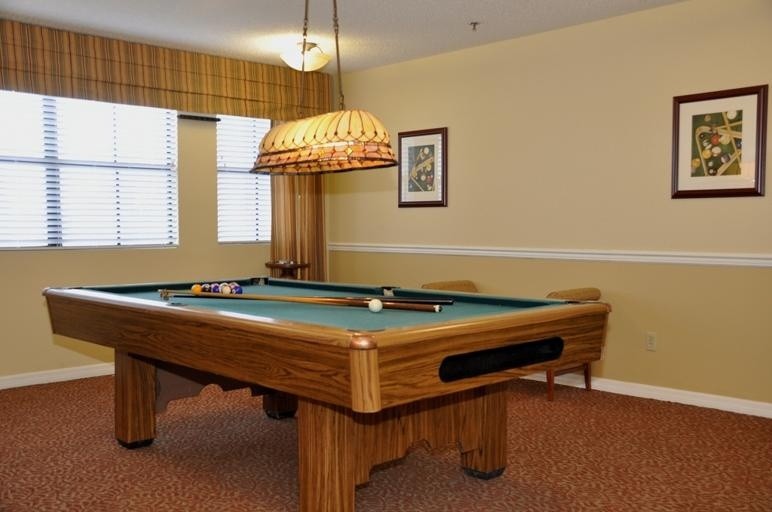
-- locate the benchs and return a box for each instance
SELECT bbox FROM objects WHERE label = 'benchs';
[423,280,600,401]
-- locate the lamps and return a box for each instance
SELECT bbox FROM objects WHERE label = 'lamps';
[249,0,399,174]
[280,43,331,72]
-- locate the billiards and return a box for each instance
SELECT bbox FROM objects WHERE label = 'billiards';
[191,282,244,297]
[691,110,741,175]
[369,299,382,314]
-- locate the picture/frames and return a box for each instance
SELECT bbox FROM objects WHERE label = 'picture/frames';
[671,84,768,198]
[398,127,447,207]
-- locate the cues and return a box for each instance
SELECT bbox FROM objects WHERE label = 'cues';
[157,287,442,313]
[160,291,454,306]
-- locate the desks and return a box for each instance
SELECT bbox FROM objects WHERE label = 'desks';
[43,276,612,512]
[265,259,310,279]
